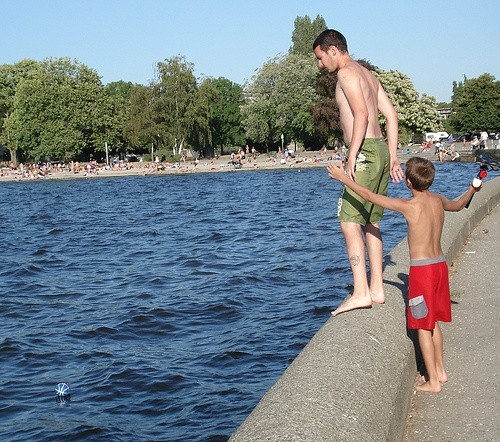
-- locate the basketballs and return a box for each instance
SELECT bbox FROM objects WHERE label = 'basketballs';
[55,383,70,396]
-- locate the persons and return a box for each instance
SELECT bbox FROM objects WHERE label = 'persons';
[0,153,202,181]
[207,143,347,169]
[408,131,500,160]
[313,29,405,316]
[326,157,478,392]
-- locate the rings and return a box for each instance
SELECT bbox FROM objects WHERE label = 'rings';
[396,170,399,172]
